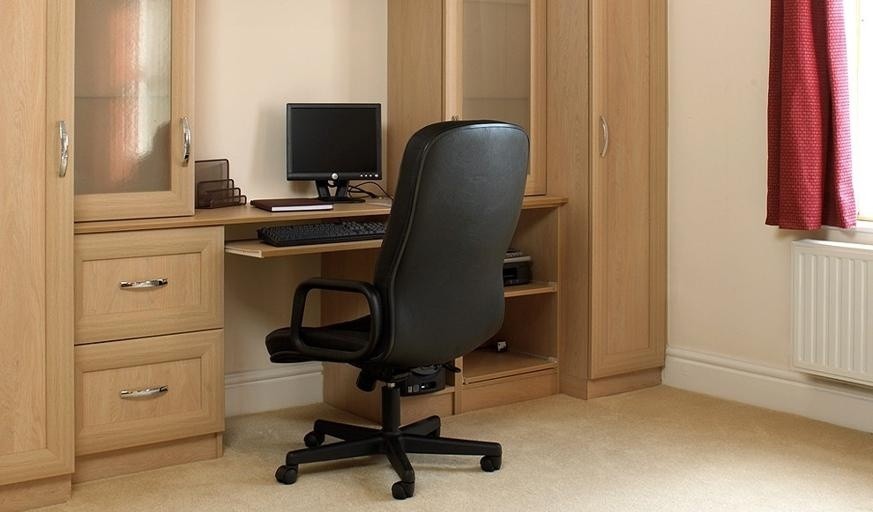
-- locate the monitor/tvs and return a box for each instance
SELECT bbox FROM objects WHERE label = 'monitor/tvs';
[286,102,382,203]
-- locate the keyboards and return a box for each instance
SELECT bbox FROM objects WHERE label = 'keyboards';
[258,222,387,247]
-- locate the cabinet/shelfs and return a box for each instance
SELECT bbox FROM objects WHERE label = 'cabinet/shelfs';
[1,2,73,493]
[547,1,667,400]
[75,0,198,221]
[389,1,550,199]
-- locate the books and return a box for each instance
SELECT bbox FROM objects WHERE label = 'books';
[250,197,333,212]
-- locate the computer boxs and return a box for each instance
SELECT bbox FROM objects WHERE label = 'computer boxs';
[396,362,446,396]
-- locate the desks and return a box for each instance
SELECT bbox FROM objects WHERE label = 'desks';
[69,191,567,489]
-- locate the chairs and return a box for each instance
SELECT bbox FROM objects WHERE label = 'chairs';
[266,122,532,496]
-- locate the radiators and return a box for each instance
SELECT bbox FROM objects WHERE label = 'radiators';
[791,234,872,394]
